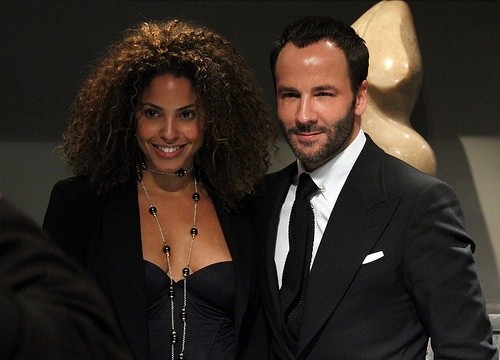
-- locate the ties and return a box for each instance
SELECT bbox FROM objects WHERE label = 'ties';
[280,173,320,340]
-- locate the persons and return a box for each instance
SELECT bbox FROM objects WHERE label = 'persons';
[233,15,497,360]
[41,16,262,360]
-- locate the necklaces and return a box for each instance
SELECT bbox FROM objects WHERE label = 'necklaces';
[131,163,200,360]
